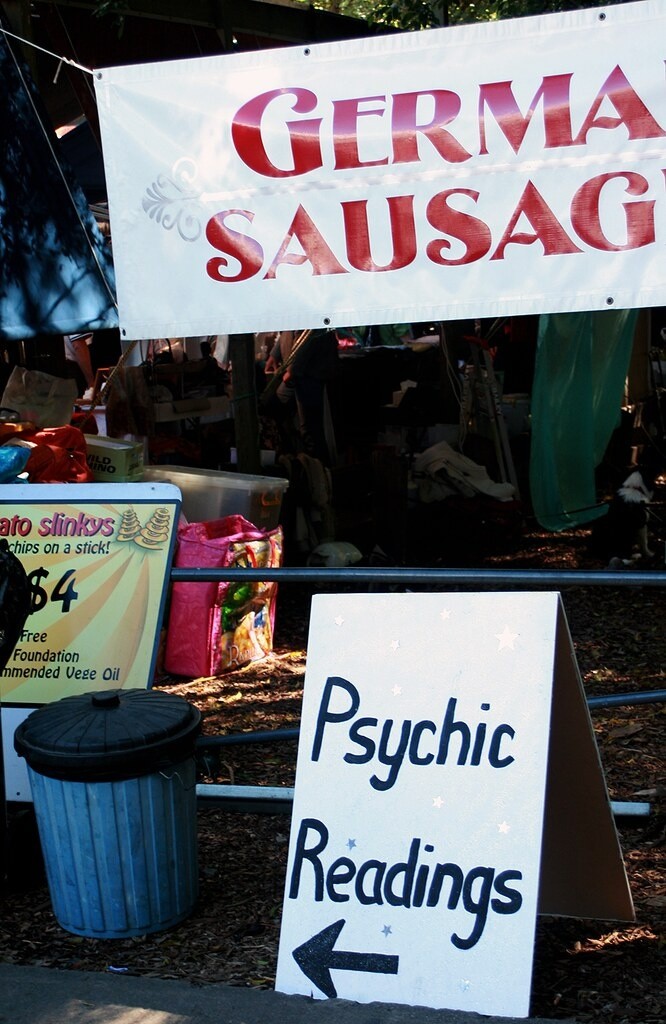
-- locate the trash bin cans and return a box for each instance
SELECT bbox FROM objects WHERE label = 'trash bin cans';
[13,688,209,939]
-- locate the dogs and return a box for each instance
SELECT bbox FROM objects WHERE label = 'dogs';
[581,464,664,569]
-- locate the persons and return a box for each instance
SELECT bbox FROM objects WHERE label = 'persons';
[262,329,333,470]
[441,319,493,406]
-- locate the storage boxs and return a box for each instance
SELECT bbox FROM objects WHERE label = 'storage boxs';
[76,432,145,483]
[141,464,291,531]
[499,399,530,431]
